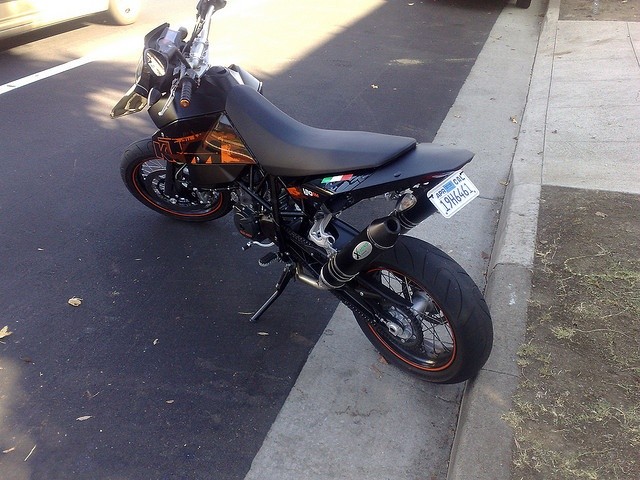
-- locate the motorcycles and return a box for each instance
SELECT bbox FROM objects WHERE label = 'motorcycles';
[109,0,492,384]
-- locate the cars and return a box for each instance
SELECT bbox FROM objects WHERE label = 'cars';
[0,1,143,40]
